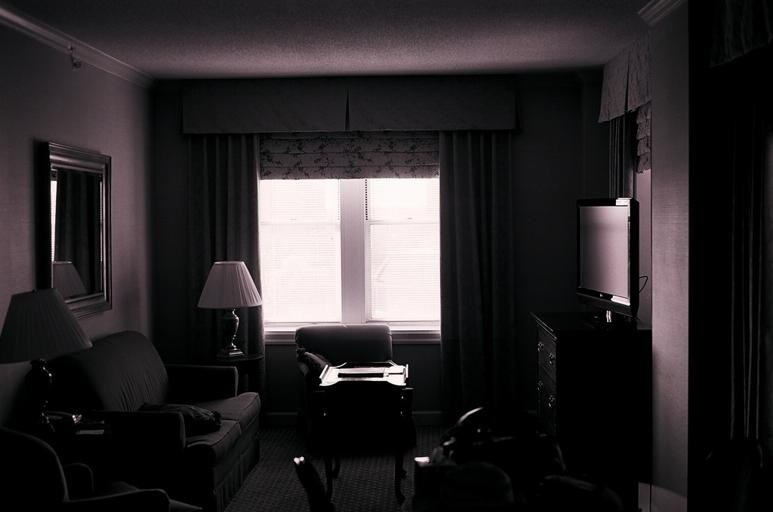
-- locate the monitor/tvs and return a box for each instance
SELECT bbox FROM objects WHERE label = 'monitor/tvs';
[573,197,640,328]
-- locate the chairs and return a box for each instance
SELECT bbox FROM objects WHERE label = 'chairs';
[291,322,419,450]
[291,455,341,511]
[0,427,205,512]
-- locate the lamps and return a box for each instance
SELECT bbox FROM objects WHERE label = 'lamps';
[51,257,89,297]
[191,260,264,357]
[0,283,95,437]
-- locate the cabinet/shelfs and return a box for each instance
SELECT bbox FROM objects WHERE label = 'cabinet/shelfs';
[524,302,652,462]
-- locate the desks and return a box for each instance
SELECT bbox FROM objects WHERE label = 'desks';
[43,423,112,473]
[200,356,268,425]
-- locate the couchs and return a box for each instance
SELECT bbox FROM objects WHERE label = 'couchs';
[71,325,267,512]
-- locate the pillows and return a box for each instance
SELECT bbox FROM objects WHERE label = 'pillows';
[137,398,222,435]
[294,345,328,372]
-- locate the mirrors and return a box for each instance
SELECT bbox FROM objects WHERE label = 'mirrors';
[37,135,115,322]
